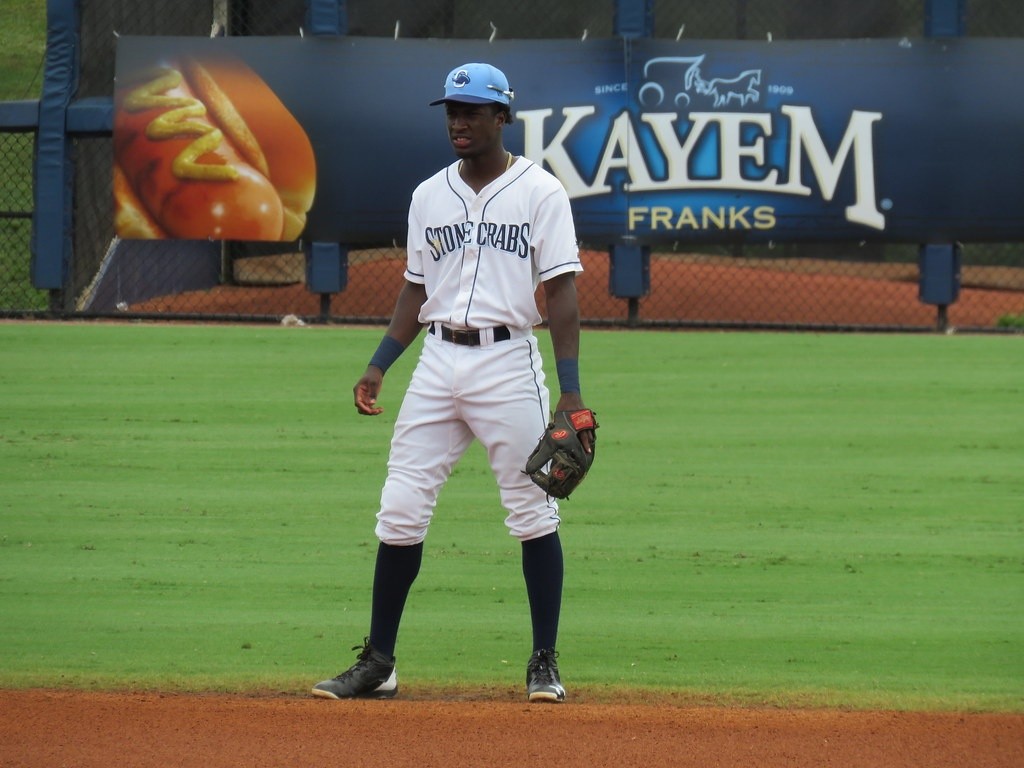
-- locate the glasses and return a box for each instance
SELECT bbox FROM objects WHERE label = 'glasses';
[487,84,514,101]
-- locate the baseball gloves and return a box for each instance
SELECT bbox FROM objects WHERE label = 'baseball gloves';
[519,408,601,505]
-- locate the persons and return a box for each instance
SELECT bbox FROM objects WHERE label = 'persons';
[310,63,600,706]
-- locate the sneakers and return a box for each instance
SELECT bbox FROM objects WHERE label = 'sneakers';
[311,636,398,700]
[526,644,566,703]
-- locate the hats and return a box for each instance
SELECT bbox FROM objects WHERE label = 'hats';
[429,63,509,108]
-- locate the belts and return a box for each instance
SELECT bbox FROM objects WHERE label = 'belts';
[429,321,510,345]
